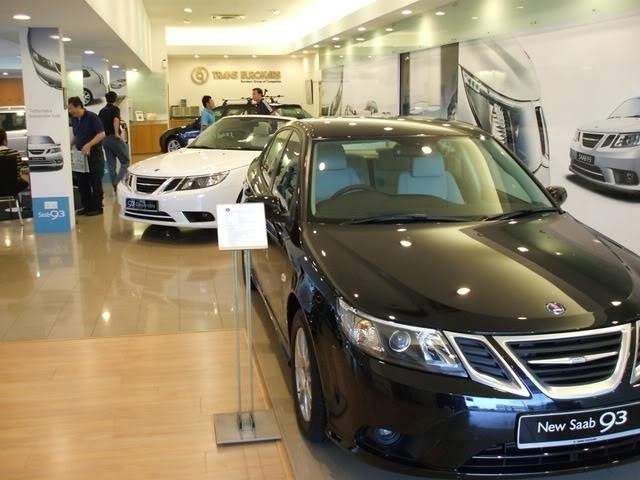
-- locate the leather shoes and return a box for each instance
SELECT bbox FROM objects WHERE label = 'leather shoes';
[77,208,87,215]
[87,208,103,216]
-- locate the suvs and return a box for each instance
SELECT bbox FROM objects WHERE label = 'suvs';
[0,104,29,165]
[158,89,315,156]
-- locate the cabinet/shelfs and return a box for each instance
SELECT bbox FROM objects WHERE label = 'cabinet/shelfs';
[129,117,195,155]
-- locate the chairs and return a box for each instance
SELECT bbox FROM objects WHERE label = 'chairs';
[308,141,469,214]
[246,121,276,149]
[0,153,29,226]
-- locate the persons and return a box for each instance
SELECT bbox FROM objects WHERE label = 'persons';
[252,88,274,116]
[0,129,29,194]
[68,96,105,216]
[98,92,130,192]
[199,96,214,132]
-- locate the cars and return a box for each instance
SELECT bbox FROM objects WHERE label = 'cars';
[320,63,343,117]
[232,112,639,479]
[26,27,62,91]
[110,78,126,89]
[83,62,106,107]
[456,37,551,187]
[565,93,640,193]
[110,113,297,238]
[26,135,66,173]
